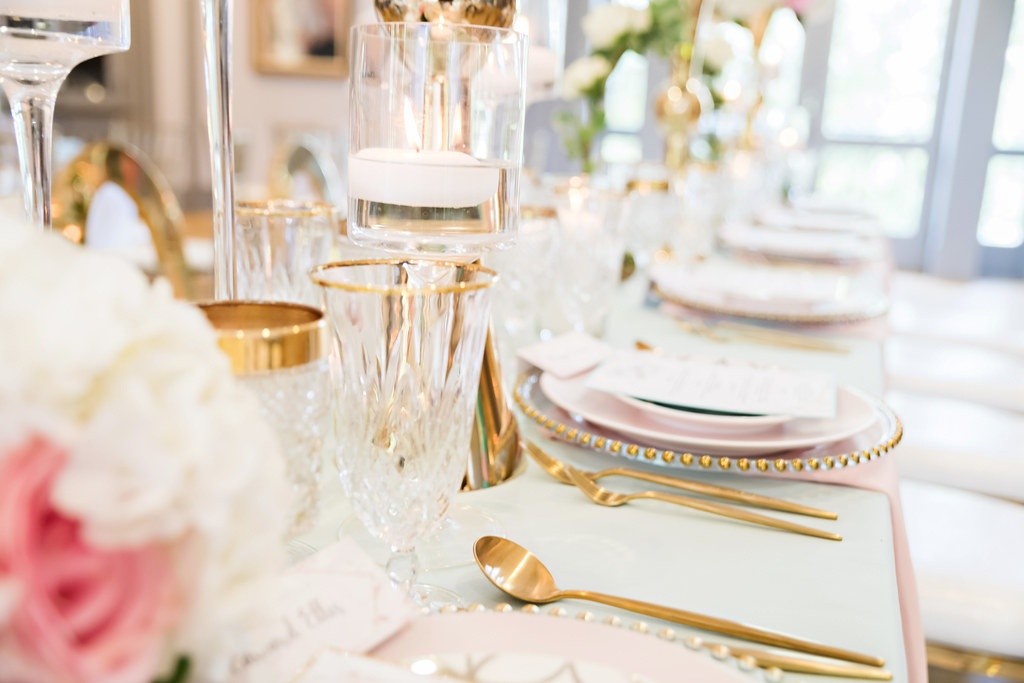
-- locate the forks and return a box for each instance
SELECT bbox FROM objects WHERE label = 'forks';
[562,462,844,542]
[513,437,836,520]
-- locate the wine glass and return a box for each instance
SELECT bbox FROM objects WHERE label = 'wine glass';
[1,0,131,238]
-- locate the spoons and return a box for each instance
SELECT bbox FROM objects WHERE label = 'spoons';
[472,536,885,667]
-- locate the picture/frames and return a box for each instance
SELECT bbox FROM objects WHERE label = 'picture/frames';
[249,0,352,79]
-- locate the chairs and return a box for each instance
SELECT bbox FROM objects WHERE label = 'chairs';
[885,270,1024,683]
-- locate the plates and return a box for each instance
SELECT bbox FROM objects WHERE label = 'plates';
[512,365,904,473]
[617,392,791,436]
[650,204,884,322]
[372,602,784,682]
[536,370,881,457]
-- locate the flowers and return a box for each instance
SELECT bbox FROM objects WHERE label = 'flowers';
[0,212,286,683]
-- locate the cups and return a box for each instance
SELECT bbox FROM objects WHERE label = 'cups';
[346,20,529,254]
[213,195,342,315]
[313,252,498,611]
[195,295,328,542]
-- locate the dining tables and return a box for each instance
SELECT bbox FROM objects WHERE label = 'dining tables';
[387,219,930,683]
